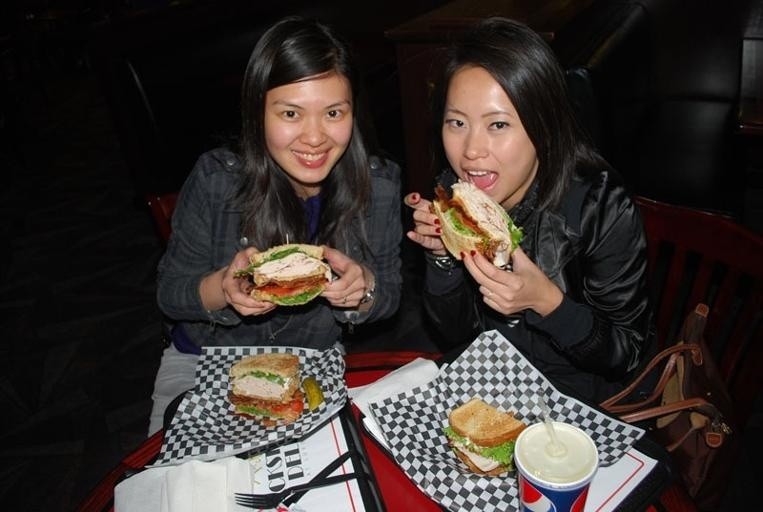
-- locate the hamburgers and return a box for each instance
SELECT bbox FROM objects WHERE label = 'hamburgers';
[427,178,527,271]
[236,242,335,308]
[225,352,305,428]
[445,397,528,478]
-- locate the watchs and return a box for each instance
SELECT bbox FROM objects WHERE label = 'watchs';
[357,261,376,315]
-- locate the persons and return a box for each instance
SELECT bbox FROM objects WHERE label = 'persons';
[403,14,661,448]
[155,18,406,438]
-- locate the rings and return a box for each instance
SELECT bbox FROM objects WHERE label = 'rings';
[342,296,347,305]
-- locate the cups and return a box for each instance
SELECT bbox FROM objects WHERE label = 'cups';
[512,419,600,512]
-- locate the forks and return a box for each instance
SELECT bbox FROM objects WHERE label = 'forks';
[234,469,372,509]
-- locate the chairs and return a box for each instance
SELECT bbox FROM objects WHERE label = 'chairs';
[630,192,763,510]
[145,191,181,245]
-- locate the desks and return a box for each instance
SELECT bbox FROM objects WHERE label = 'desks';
[386,0,594,202]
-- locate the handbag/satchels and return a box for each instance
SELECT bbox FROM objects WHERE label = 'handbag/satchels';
[656,304,733,501]
